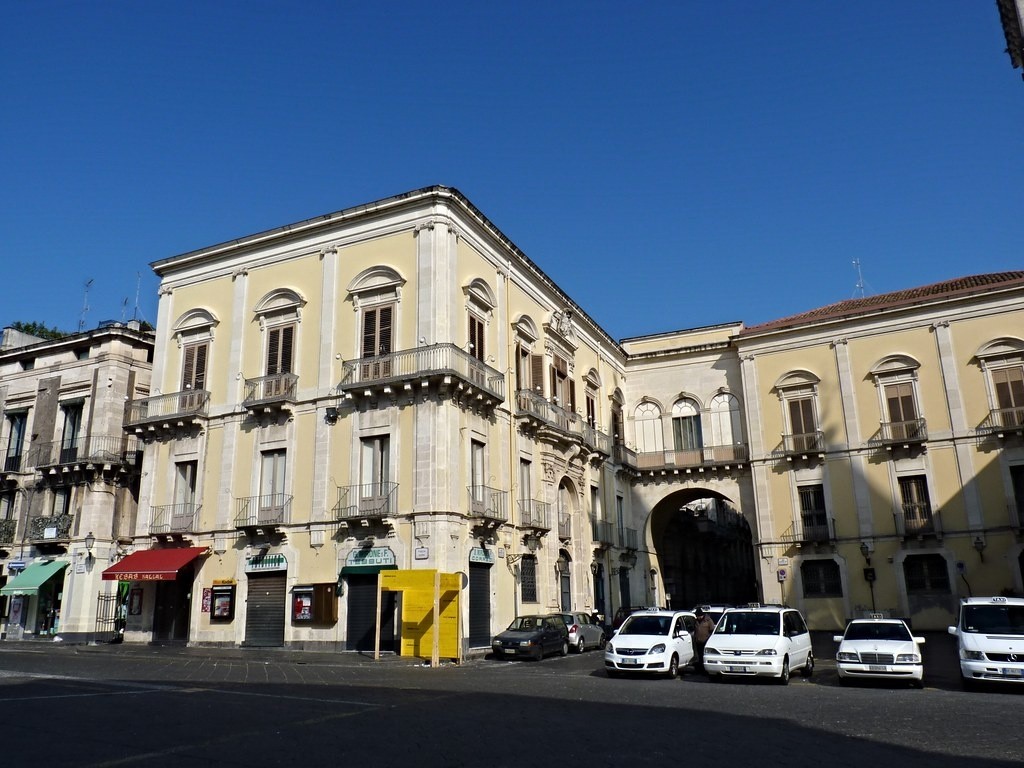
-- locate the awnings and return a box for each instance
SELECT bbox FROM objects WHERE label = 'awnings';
[0,561,67,596]
[102,546,209,580]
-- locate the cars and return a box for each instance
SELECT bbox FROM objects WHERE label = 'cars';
[492,615,569,662]
[612,606,647,635]
[834,613,925,689]
[691,605,729,633]
[703,602,815,685]
[604,606,699,680]
[948,597,1024,685]
[548,612,606,655]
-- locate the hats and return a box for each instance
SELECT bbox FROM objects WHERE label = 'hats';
[694,608,704,616]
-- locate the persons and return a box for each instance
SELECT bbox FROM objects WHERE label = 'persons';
[694,608,715,652]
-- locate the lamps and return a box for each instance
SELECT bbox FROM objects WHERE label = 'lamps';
[235,371,245,381]
[532,387,618,440]
[859,542,871,566]
[505,532,540,568]
[334,337,514,375]
[255,542,271,550]
[325,407,341,420]
[124,383,193,402]
[974,537,985,563]
[86,533,97,564]
[586,560,598,576]
[358,535,376,547]
[553,557,564,581]
